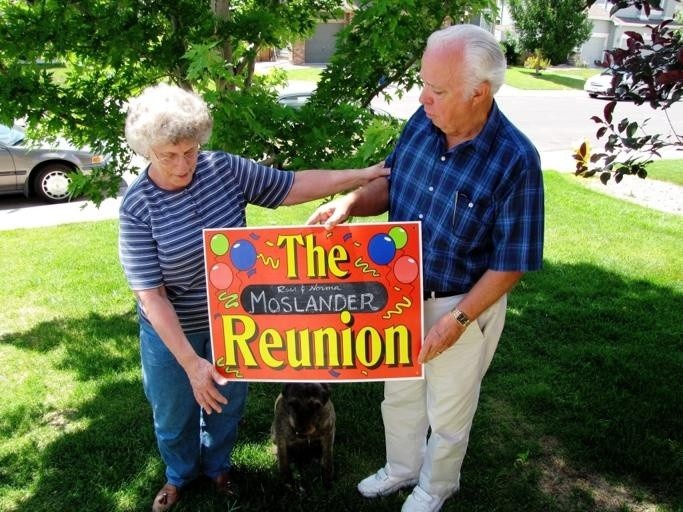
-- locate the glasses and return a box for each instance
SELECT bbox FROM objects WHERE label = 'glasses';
[151,146,203,166]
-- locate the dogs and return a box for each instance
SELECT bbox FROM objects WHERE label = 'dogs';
[270,383,336,491]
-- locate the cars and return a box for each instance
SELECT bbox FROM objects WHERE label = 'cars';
[584,62,654,101]
[0,118,113,204]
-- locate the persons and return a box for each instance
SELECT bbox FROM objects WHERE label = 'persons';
[111,80,397,510]
[299,23,545,512]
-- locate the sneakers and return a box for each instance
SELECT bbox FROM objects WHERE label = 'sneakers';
[357,468,420,499]
[212,469,241,500]
[152,482,179,512]
[400,476,460,512]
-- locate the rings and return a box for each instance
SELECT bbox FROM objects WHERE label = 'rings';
[436,351,443,356]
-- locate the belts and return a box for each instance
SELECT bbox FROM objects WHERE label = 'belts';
[422,288,465,302]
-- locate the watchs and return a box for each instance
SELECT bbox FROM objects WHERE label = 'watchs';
[448,304,475,333]
